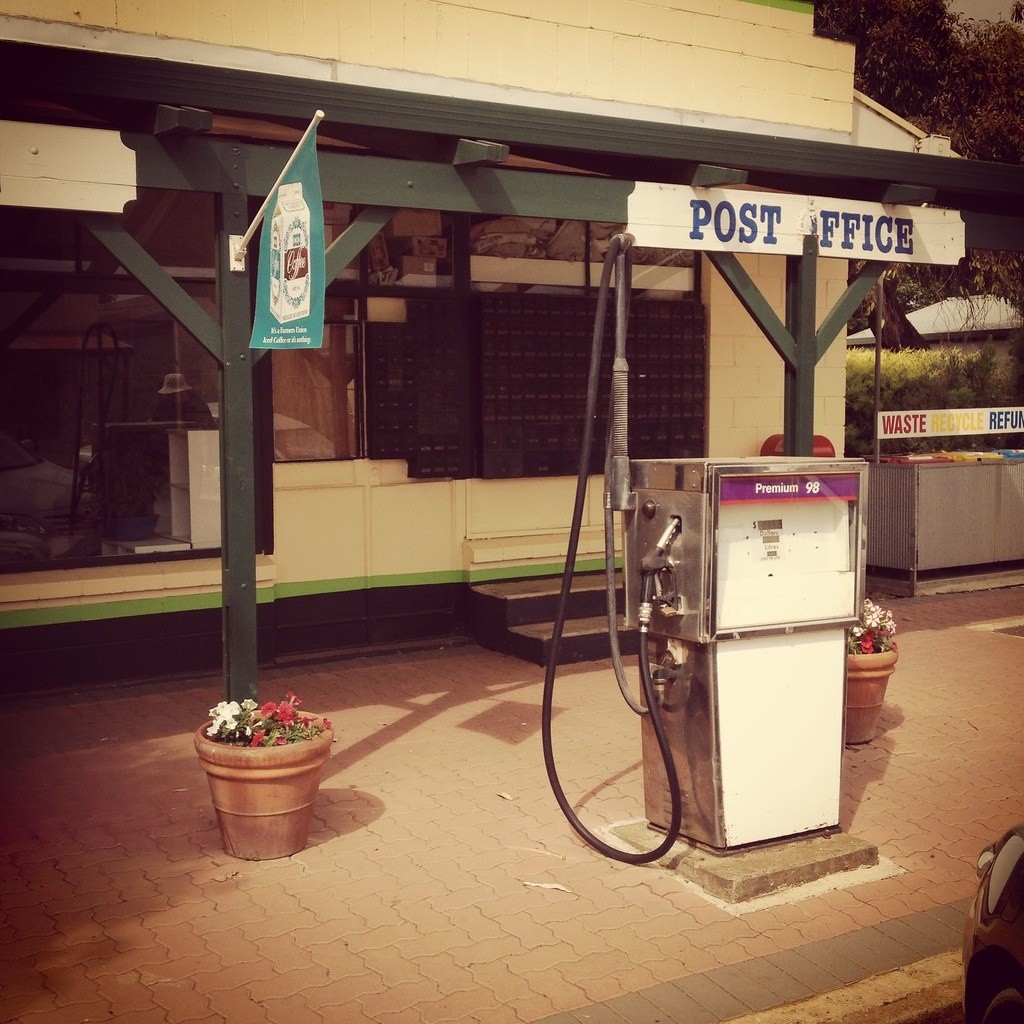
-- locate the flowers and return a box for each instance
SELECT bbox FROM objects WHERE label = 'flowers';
[848,600,898,653]
[205,690,332,747]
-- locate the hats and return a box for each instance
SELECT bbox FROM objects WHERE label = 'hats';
[157,373,192,394]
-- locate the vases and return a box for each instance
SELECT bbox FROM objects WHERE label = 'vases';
[845,644,899,744]
[193,710,332,861]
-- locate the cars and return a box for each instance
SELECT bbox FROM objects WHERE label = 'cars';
[1,425,99,573]
[205,402,338,462]
[961,826,1024,1024]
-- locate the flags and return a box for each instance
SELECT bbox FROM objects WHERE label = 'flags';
[248,123,326,350]
